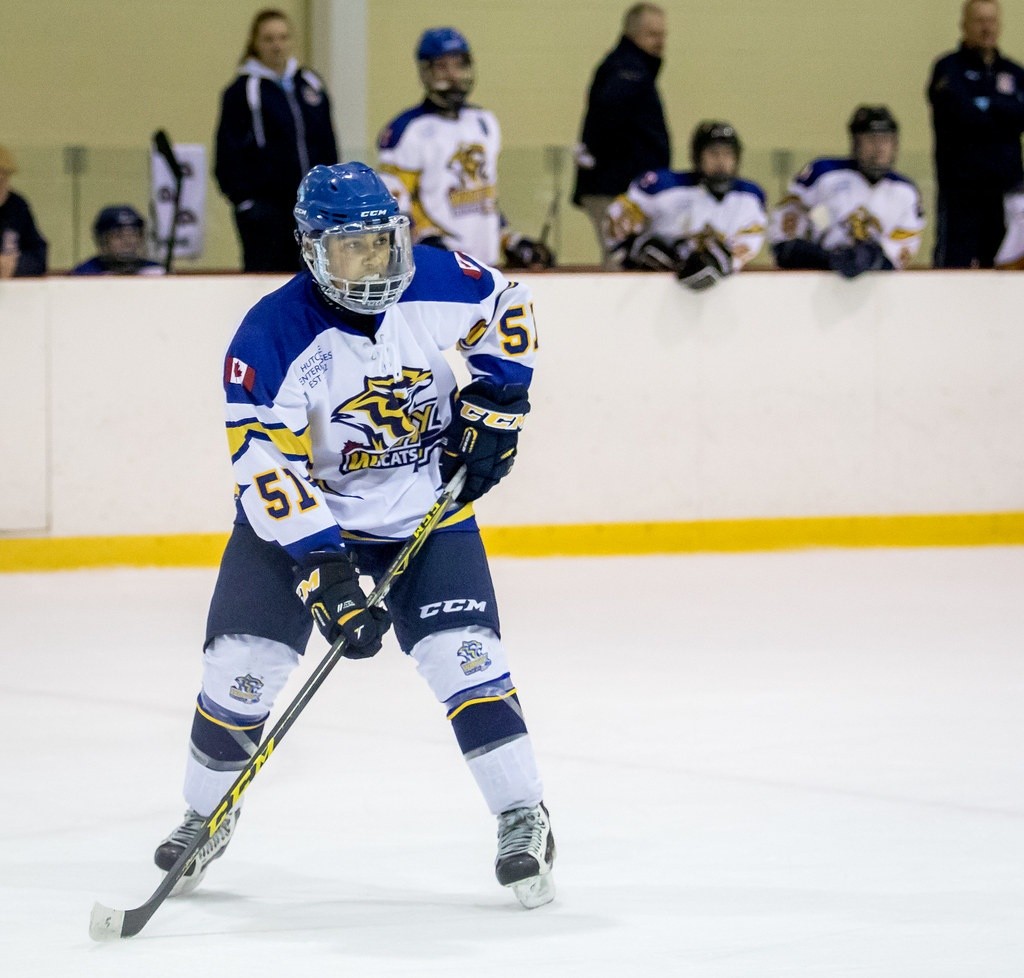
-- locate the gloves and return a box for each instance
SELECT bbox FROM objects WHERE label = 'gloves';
[608,231,681,273]
[832,240,885,278]
[413,226,462,250]
[293,544,392,659]
[503,232,556,270]
[440,381,532,504]
[675,235,732,291]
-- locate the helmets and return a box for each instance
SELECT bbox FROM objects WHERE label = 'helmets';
[849,106,897,135]
[93,205,144,236]
[689,118,744,159]
[293,163,402,235]
[416,28,469,60]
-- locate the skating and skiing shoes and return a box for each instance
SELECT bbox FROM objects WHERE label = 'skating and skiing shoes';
[494,801,557,887]
[153,811,228,877]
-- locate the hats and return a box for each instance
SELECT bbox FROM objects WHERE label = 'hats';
[0,145,16,172]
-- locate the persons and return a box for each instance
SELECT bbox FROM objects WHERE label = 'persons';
[925,0,1024,270]
[375,26,557,271]
[599,119,768,289]
[770,104,926,278]
[60,205,172,275]
[155,161,555,885]
[0,147,49,276]
[215,9,340,274]
[570,3,672,272]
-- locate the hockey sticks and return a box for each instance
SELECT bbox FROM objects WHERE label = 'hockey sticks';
[155,129,184,276]
[87,464,468,939]
[538,142,594,245]
